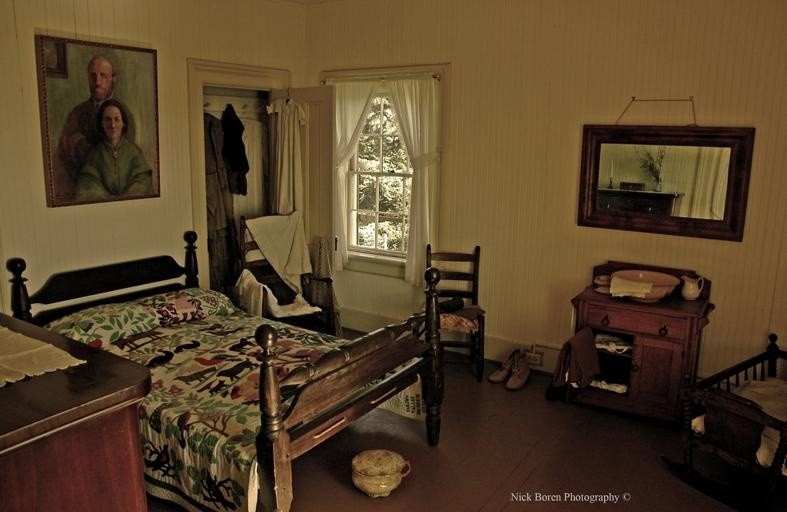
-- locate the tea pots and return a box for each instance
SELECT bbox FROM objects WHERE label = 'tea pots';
[682,275,704,303]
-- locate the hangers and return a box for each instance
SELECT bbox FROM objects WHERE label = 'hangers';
[270,86,306,116]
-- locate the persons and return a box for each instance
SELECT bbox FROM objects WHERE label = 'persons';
[56,55,137,183]
[71,98,154,200]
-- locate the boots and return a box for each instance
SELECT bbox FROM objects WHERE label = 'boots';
[487,349,520,384]
[505,352,532,392]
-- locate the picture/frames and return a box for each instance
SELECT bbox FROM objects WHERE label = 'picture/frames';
[576,123,757,243]
[32,33,161,208]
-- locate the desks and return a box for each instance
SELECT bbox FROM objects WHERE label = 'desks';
[597,188,685,216]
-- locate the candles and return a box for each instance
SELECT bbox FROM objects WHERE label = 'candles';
[611,159,614,177]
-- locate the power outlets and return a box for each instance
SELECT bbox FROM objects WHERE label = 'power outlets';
[526,352,541,365]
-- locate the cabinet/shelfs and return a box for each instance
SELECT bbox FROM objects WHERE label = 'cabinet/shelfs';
[566,260,712,425]
[0,312,152,512]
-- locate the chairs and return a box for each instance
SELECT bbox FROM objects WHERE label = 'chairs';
[239,209,336,335]
[426,244,486,383]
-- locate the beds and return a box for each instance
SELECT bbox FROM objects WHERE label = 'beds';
[5,231,446,512]
[680,333,787,512]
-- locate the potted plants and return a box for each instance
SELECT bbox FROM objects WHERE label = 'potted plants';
[631,144,669,193]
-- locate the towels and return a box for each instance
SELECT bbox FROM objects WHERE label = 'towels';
[610,276,651,299]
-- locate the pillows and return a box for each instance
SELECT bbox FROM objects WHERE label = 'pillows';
[44,301,160,348]
[134,288,234,326]
